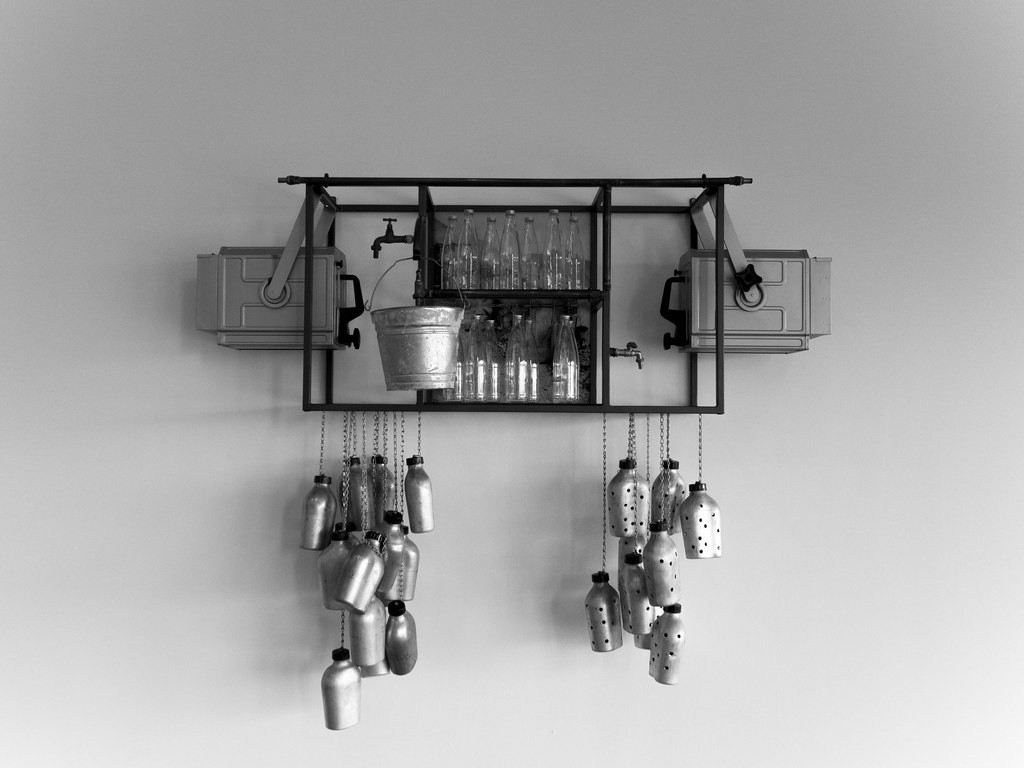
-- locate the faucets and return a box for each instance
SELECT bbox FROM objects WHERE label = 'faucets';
[369,217,416,260]
[611,340,645,370]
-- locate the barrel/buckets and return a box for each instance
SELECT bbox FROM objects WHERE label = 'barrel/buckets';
[363,255,471,391]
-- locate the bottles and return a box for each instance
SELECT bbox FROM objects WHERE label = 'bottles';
[442,312,581,404]
[481,216,502,290]
[499,209,520,289]
[520,216,542,290]
[543,208,565,291]
[565,217,585,290]
[457,208,481,289]
[584,456,723,686]
[440,215,460,290]
[299,454,435,731]
